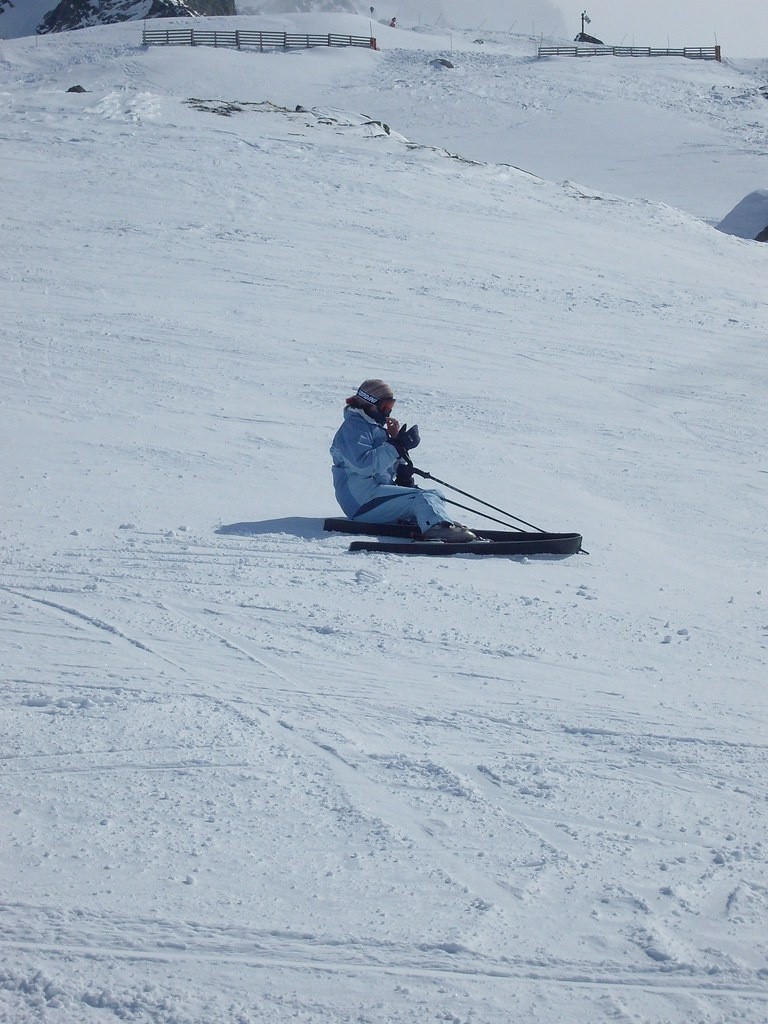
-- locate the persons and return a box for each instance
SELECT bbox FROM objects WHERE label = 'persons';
[329,378,477,544]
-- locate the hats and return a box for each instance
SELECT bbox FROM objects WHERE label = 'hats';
[346,379,393,411]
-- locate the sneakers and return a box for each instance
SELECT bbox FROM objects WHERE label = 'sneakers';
[424,521,476,542]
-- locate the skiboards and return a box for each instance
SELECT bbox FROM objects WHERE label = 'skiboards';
[322,514,586,558]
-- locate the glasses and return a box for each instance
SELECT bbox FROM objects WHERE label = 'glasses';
[377,399,395,417]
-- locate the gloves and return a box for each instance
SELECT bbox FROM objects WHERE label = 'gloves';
[395,464,415,487]
[396,423,420,451]
